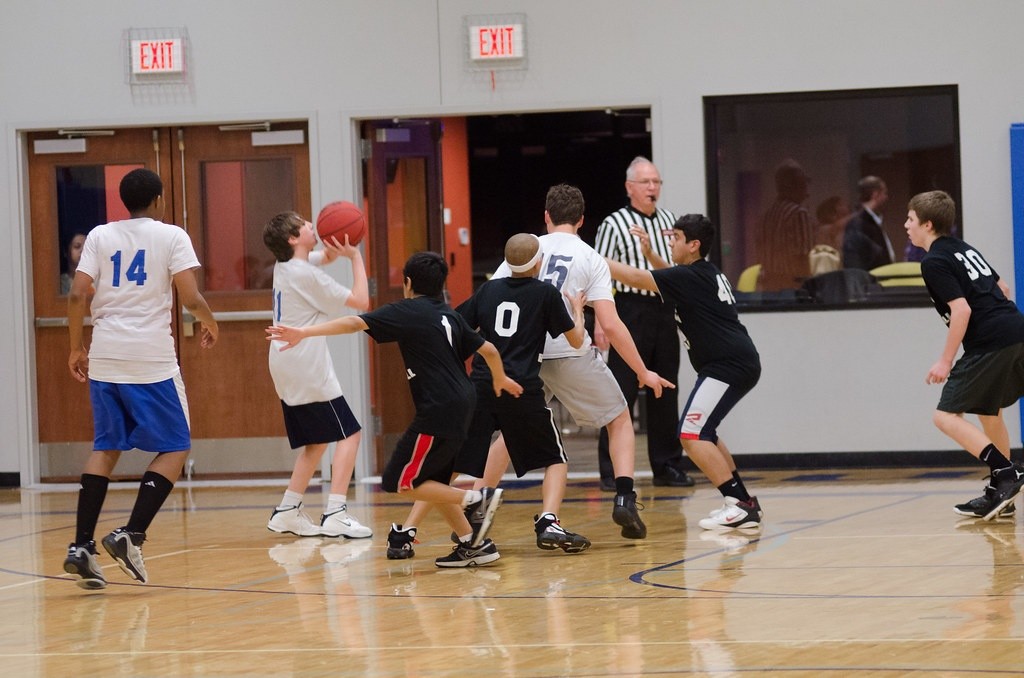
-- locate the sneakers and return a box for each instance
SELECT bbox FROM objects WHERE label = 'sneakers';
[121,604,149,673]
[102,526,149,584]
[463,487,503,548]
[532,513,592,554]
[954,516,1021,555]
[437,570,501,593]
[64,540,108,589]
[320,506,374,538]
[699,495,764,527]
[653,466,696,487]
[72,598,108,646]
[386,563,417,595]
[269,537,324,567]
[320,539,373,567]
[599,475,618,491]
[386,522,417,559]
[267,501,323,536]
[983,460,1024,522]
[952,485,1017,517]
[435,538,501,568]
[700,528,760,555]
[613,491,648,539]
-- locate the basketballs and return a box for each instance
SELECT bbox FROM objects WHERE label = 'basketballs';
[316,202,367,247]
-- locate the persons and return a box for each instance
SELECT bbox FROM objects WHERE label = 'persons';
[801,244,872,303]
[384,233,590,560]
[65,169,219,590]
[260,211,373,540]
[601,215,763,530]
[724,158,854,302]
[904,190,1024,521]
[60,234,93,293]
[594,155,695,490]
[841,175,896,269]
[266,251,506,569]
[451,184,677,544]
